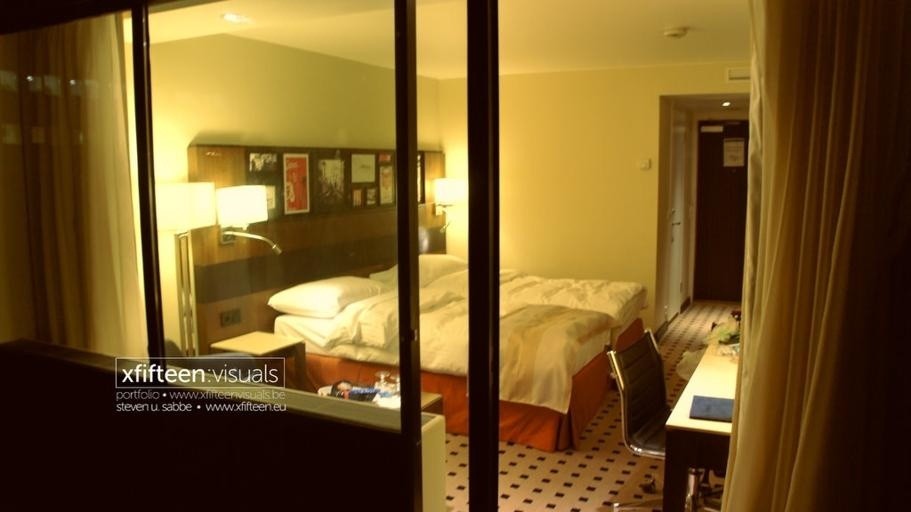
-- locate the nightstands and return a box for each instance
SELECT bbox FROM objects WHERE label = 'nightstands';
[212,328,307,390]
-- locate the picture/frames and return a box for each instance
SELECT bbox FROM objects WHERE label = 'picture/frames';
[346,150,381,191]
[280,151,316,217]
[245,147,278,179]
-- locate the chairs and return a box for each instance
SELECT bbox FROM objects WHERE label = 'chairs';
[608,327,700,512]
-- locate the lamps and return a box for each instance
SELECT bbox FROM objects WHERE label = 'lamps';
[432,178,462,232]
[215,184,270,243]
[224,230,284,257]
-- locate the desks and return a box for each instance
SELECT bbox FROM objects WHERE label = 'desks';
[663,322,736,512]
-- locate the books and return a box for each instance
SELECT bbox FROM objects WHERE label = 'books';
[690,395,733,422]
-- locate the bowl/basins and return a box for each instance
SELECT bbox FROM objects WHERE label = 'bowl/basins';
[349,388,379,401]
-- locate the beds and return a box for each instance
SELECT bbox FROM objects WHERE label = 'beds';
[175,206,645,454]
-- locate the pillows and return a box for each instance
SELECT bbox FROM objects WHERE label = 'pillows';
[267,276,387,319]
[370,252,466,288]
